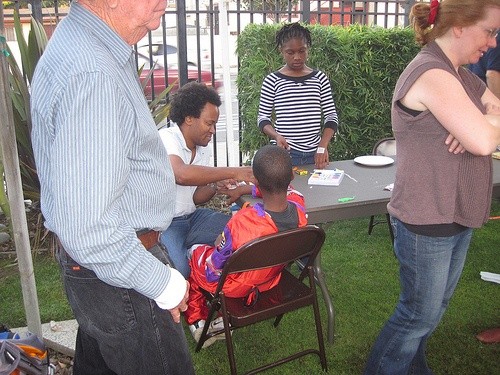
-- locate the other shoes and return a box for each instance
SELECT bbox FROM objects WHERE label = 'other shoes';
[209,316,233,338]
[189,320,216,349]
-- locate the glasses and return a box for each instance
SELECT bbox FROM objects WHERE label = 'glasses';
[458,15,500,39]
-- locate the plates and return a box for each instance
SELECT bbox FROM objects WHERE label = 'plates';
[353,155,394,166]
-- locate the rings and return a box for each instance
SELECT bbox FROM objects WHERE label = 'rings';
[179,304,188,312]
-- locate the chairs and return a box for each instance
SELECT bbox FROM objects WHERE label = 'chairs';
[194,224,329,375]
[368,137,396,244]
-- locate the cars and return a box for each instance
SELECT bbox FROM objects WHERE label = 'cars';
[138,41,209,68]
[130,50,223,104]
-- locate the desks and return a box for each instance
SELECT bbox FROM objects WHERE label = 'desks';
[238,153,500,346]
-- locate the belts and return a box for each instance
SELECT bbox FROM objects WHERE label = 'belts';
[138,230,160,248]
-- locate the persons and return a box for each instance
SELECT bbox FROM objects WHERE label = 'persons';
[258,22,338,272]
[155,82,259,279]
[469,26,500,101]
[365,0,500,375]
[475,328,500,343]
[30,0,194,375]
[184,146,308,349]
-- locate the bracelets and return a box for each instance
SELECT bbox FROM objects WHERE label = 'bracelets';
[211,182,219,192]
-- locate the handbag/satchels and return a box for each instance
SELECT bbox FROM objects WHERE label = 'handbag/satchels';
[0,322,20,340]
[0,330,56,375]
